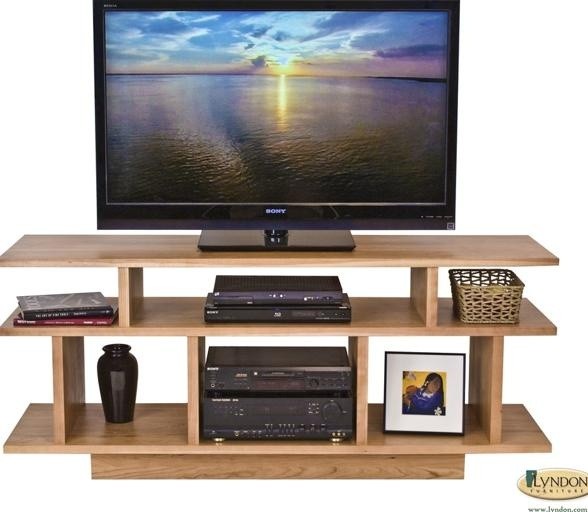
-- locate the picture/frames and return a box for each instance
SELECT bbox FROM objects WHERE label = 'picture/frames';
[383,351,466,435]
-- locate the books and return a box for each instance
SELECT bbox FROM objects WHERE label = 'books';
[16,291,114,320]
[12,298,118,326]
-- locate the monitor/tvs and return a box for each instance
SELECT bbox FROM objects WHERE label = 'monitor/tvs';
[92,0,460,251]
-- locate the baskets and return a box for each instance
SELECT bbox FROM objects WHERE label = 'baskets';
[448,269,525,324]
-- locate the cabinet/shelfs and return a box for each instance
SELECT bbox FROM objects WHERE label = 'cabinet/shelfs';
[0,234,560,480]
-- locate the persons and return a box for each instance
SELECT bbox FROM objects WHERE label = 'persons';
[402,372,444,414]
